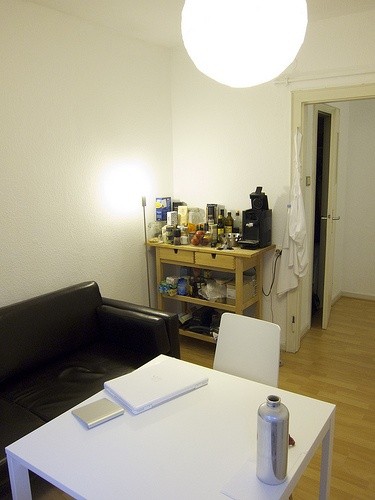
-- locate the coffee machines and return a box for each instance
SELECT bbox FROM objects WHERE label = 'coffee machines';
[238,186,272,250]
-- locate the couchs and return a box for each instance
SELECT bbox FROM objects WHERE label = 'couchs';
[0,281,181,500]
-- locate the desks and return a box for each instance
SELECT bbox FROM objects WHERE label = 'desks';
[11,352,337,500]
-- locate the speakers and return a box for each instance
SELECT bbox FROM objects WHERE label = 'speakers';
[251,195,269,210]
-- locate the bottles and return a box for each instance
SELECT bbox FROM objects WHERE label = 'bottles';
[166,224,175,244]
[220,209,226,224]
[224,211,233,233]
[209,314,219,336]
[218,215,225,243]
[232,211,242,234]
[173,207,218,247]
[188,276,197,297]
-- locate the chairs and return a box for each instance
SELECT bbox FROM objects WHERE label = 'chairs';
[214,313,281,386]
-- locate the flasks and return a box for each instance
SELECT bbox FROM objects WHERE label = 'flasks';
[255,395,290,485]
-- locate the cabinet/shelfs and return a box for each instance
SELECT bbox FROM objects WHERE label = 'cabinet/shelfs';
[145,235,276,345]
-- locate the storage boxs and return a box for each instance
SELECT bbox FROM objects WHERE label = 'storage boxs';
[156,197,171,221]
[226,276,256,305]
